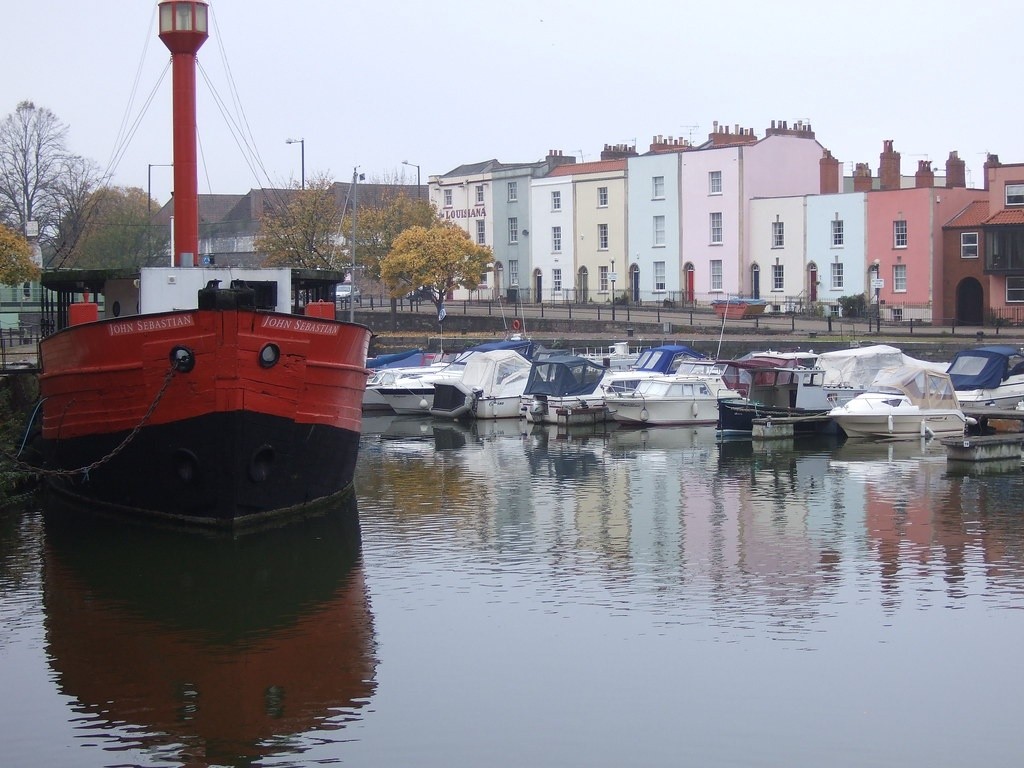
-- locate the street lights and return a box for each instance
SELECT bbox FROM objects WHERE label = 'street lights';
[347,165,366,322]
[403,160,421,197]
[610,256,618,320]
[874,259,883,332]
[287,139,304,190]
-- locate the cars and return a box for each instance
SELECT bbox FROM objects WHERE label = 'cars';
[335,285,361,304]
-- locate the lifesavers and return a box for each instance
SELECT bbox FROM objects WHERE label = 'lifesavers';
[514,320,520,330]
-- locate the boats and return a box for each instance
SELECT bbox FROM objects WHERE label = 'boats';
[362,341,953,447]
[711,299,769,321]
[38,480,377,768]
[11,0,373,534]
[821,367,976,443]
[929,345,1024,415]
